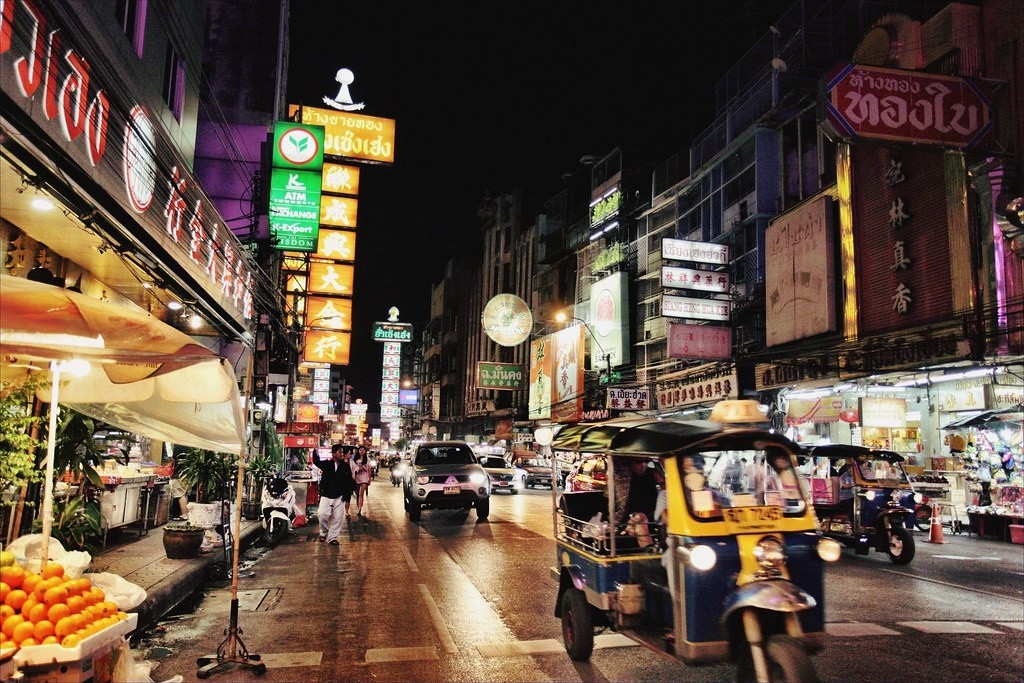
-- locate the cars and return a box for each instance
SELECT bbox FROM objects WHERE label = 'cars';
[565,453,607,494]
[477,454,522,494]
[501,450,561,488]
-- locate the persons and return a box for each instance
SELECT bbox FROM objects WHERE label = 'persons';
[836,460,888,515]
[312,444,372,546]
[543,452,584,485]
[596,449,732,549]
[364,450,406,487]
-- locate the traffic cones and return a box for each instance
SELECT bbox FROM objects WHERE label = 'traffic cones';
[922,502,951,544]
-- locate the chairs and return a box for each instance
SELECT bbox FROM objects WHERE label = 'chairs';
[563,492,638,551]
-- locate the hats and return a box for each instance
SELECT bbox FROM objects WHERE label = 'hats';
[329,444,343,452]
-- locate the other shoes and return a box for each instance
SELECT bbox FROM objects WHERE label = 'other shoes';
[329,539,340,545]
[320,535,326,543]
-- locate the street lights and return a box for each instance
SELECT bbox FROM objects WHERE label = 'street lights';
[557,313,612,419]
[38,351,90,568]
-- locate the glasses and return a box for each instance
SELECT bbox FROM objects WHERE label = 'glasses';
[634,458,651,464]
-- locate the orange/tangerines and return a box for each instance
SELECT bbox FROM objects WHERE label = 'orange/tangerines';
[0,550,127,651]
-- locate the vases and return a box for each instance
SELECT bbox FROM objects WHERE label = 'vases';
[164,524,205,559]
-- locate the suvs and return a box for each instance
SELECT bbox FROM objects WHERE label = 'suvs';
[403,440,491,522]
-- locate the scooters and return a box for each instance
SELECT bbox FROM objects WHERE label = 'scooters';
[369,458,379,480]
[392,464,403,489]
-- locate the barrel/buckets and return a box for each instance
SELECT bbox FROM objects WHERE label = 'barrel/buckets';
[1009,525,1024,544]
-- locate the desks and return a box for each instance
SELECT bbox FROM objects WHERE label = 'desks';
[968,507,1024,538]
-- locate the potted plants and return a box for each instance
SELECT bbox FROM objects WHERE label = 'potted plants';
[243,457,271,521]
[181,448,238,551]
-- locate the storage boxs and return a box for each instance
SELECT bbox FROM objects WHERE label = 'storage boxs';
[19,636,127,683]
[1008,525,1024,544]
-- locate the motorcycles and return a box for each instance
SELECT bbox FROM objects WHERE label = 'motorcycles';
[549,397,833,683]
[793,444,919,566]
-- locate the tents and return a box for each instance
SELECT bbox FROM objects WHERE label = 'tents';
[0,275,247,659]
